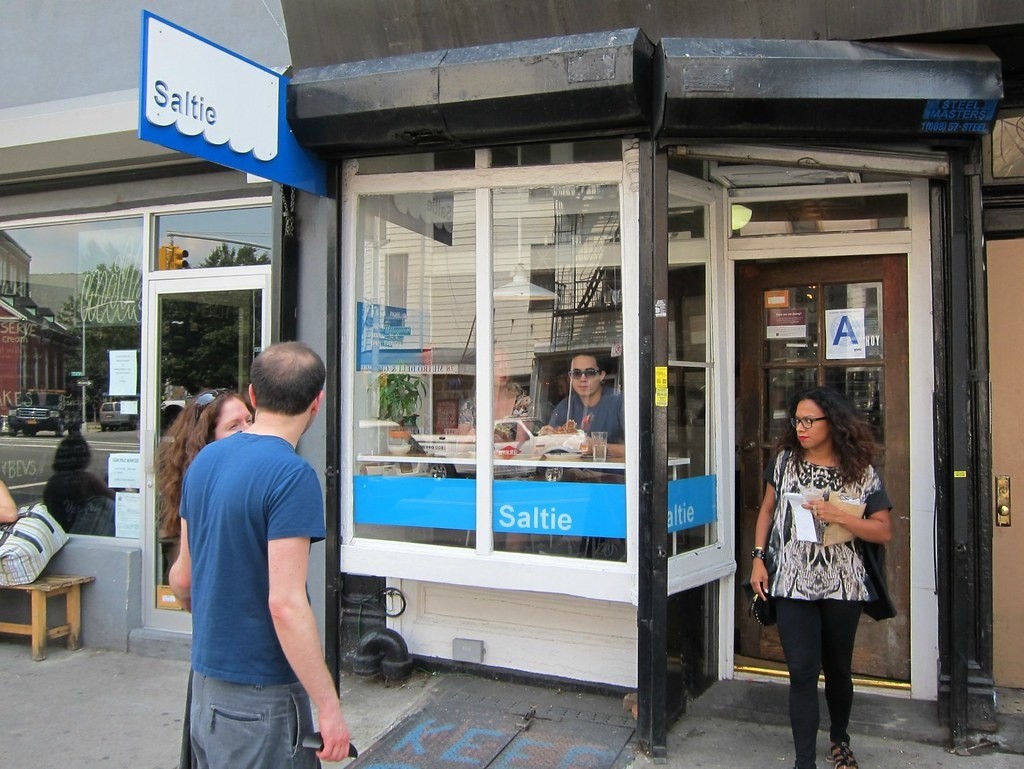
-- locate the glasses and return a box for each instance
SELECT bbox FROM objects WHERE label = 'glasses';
[194,388,229,428]
[568,370,600,379]
[790,417,827,428]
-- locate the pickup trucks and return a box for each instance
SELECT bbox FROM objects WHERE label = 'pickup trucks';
[417,418,590,481]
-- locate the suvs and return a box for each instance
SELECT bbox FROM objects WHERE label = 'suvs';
[99,402,136,431]
[8,394,81,437]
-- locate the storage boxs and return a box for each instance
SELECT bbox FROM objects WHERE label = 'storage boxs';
[157,586,184,611]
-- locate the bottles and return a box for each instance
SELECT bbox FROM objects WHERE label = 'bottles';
[387,430,412,455]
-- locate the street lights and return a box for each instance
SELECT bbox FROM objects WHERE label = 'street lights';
[81,299,136,421]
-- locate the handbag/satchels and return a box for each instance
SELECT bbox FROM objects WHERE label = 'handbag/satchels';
[0,502,69,586]
[70,496,115,536]
[742,548,776,626]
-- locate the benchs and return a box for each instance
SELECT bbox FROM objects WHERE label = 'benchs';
[0,573,96,661]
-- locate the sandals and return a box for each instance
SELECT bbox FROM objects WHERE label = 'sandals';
[831,742,858,769]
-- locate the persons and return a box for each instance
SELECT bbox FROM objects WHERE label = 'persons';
[0,480,19,523]
[169,341,351,769]
[152,388,254,769]
[462,349,534,552]
[46,433,116,533]
[546,351,625,555]
[751,385,894,769]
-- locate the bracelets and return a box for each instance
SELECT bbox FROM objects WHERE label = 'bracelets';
[751,546,766,560]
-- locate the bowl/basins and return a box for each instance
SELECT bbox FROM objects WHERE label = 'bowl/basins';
[412,433,589,459]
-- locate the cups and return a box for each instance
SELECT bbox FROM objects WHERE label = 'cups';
[801,488,824,503]
[591,432,607,463]
[445,428,469,458]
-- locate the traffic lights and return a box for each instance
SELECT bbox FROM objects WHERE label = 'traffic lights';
[170,246,188,269]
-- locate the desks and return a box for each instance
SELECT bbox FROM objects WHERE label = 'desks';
[356,452,691,557]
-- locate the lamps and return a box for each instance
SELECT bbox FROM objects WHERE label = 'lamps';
[491,145,559,300]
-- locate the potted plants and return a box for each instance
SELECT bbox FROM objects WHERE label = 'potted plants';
[366,372,428,435]
